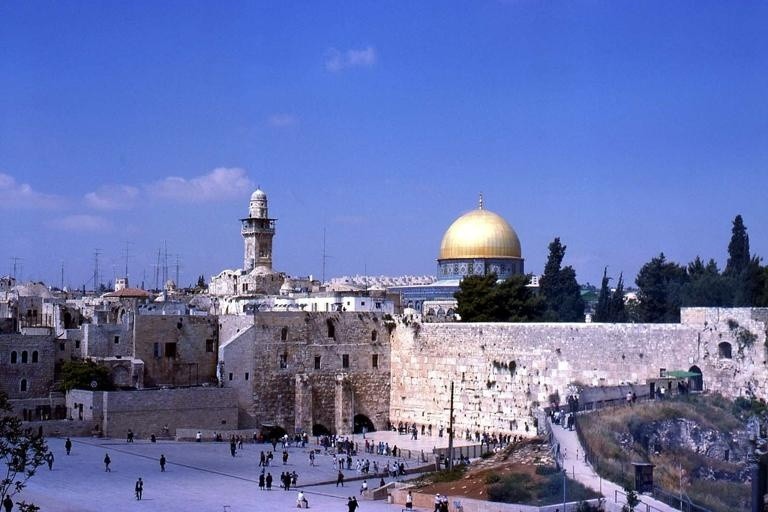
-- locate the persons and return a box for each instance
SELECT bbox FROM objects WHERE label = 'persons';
[46,452,54,470]
[655,380,689,401]
[127,429,132,442]
[150,433,156,444]
[104,453,111,472]
[135,477,143,500]
[625,391,632,407]
[64,438,71,456]
[632,392,637,403]
[549,395,579,431]
[159,455,166,472]
[3,494,13,512]
[214,423,526,512]
[196,431,201,442]
